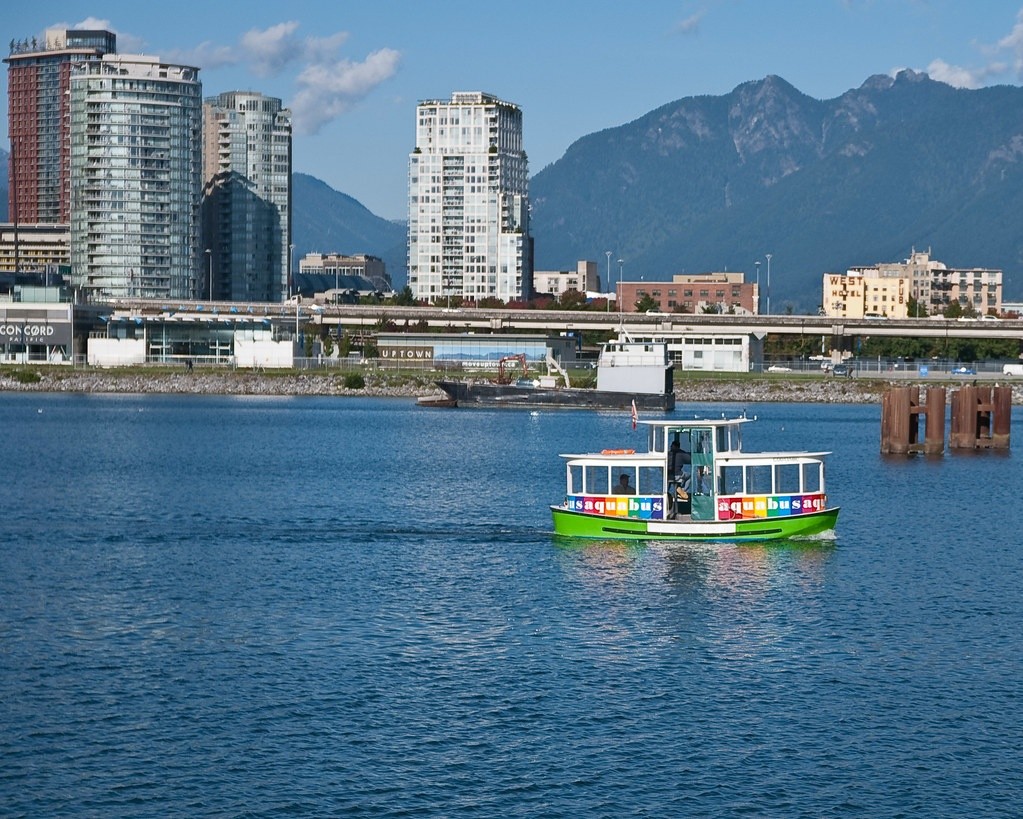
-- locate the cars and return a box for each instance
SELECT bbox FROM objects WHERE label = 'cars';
[862,312,1000,321]
[1002,358,1023,377]
[832,364,848,378]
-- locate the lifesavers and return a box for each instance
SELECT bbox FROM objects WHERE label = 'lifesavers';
[601,448,635,454]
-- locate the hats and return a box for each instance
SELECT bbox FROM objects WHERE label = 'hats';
[620,474,630,479]
[673,440,681,447]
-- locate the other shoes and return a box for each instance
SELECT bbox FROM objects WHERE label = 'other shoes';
[677,487,688,499]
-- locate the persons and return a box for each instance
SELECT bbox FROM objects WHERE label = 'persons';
[668,440,691,509]
[613,474,635,494]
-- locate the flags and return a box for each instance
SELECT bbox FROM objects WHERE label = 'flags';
[632,399,638,429]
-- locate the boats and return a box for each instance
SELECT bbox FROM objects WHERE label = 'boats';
[548,409,841,543]
[412,258,676,417]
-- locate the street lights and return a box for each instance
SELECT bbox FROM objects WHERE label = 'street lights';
[205,244,773,316]
